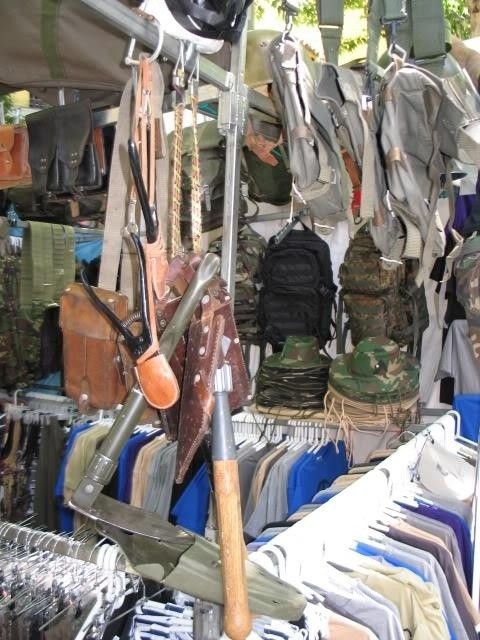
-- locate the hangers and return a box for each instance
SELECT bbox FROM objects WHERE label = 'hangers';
[0,522,170,639]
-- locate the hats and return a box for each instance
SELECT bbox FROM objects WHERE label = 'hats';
[253,334,421,426]
